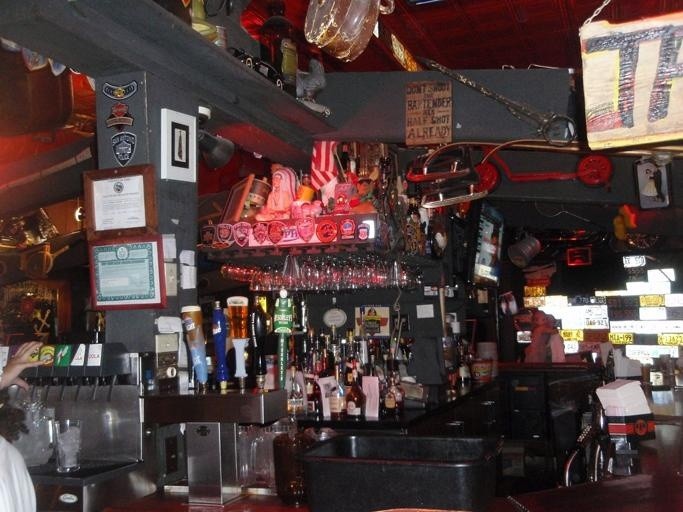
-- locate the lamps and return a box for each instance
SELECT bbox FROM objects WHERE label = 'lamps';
[196,128,236,171]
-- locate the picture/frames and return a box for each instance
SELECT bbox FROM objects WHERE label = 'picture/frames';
[159,107,197,184]
[219,172,255,225]
[633,156,674,212]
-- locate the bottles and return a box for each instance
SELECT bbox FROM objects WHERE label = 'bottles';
[286,324,410,420]
[457,344,471,388]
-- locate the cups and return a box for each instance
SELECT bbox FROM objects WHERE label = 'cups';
[53,416,82,474]
[181,304,203,341]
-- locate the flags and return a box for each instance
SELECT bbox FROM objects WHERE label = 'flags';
[308,140,342,193]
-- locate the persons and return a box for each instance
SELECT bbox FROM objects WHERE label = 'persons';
[641,168,658,202]
[1,338,51,395]
[259,170,293,220]
[512,308,568,363]
[647,164,666,203]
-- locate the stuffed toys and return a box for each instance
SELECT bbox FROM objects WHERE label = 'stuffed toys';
[349,178,380,213]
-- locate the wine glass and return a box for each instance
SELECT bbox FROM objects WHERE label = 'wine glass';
[248,254,407,294]
